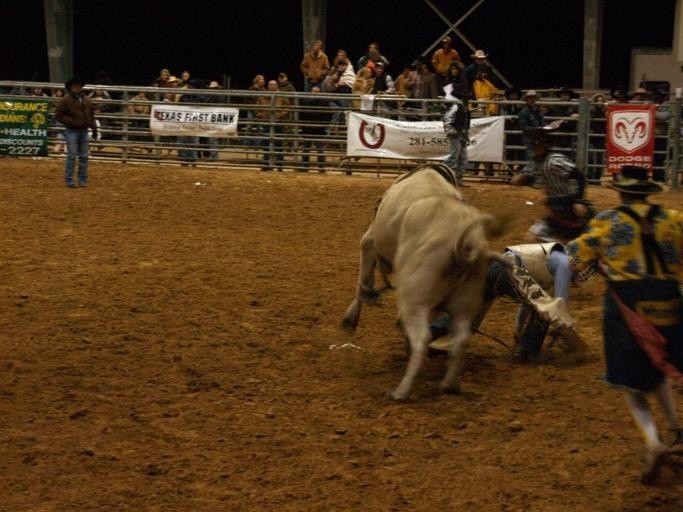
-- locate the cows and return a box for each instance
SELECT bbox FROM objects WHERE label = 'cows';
[340,162,513,405]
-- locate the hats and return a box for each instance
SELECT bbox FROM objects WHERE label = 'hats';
[471,50,487,59]
[606,165,664,195]
[505,87,575,100]
[208,82,220,88]
[65,77,85,91]
[166,76,180,82]
[627,87,653,96]
[411,55,425,66]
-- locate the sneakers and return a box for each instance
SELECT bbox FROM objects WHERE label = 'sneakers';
[640,443,670,485]
[513,349,540,364]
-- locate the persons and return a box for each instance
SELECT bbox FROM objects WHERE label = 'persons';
[55,77,98,188]
[404,239,600,364]
[509,128,597,244]
[244,37,673,187]
[10,87,111,138]
[128,91,149,141]
[154,69,223,166]
[563,164,683,485]
